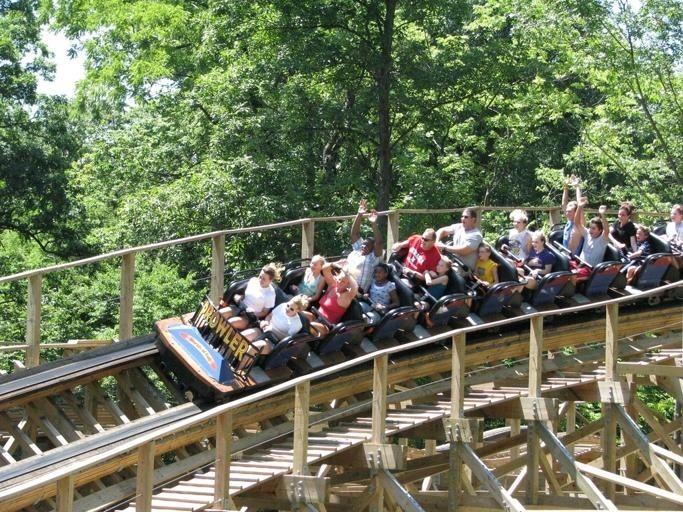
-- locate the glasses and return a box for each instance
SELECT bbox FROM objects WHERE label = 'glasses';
[461,215,471,218]
[286,304,295,312]
[422,237,431,242]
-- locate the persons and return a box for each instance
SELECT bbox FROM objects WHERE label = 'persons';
[666,204,683,256]
